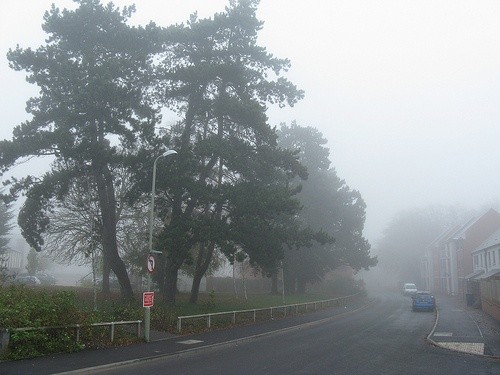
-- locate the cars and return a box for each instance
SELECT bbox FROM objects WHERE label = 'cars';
[401,283,418,297]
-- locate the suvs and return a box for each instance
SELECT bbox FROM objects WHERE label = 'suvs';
[411,290,435,312]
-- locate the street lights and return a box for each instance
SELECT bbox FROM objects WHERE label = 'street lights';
[144,149,184,336]
